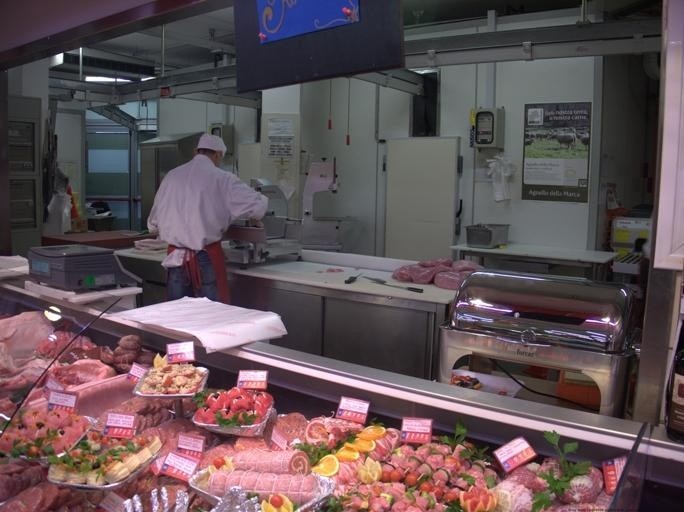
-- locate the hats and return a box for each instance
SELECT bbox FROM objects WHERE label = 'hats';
[198,134,226,156]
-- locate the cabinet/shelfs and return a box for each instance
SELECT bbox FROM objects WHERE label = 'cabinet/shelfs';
[140,132,203,232]
[9,96,42,258]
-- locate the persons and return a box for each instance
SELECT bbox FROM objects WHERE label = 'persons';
[145,130,270,307]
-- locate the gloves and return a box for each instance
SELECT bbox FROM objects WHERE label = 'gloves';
[248,219,264,227]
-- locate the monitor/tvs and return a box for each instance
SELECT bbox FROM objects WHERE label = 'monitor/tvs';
[232,0,407,94]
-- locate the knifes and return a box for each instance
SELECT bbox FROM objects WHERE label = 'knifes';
[363,275,424,293]
[345,271,362,284]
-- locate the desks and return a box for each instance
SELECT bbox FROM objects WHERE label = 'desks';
[448,244,621,283]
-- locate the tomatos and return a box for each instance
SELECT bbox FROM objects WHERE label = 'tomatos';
[213,458,226,469]
[270,495,284,507]
